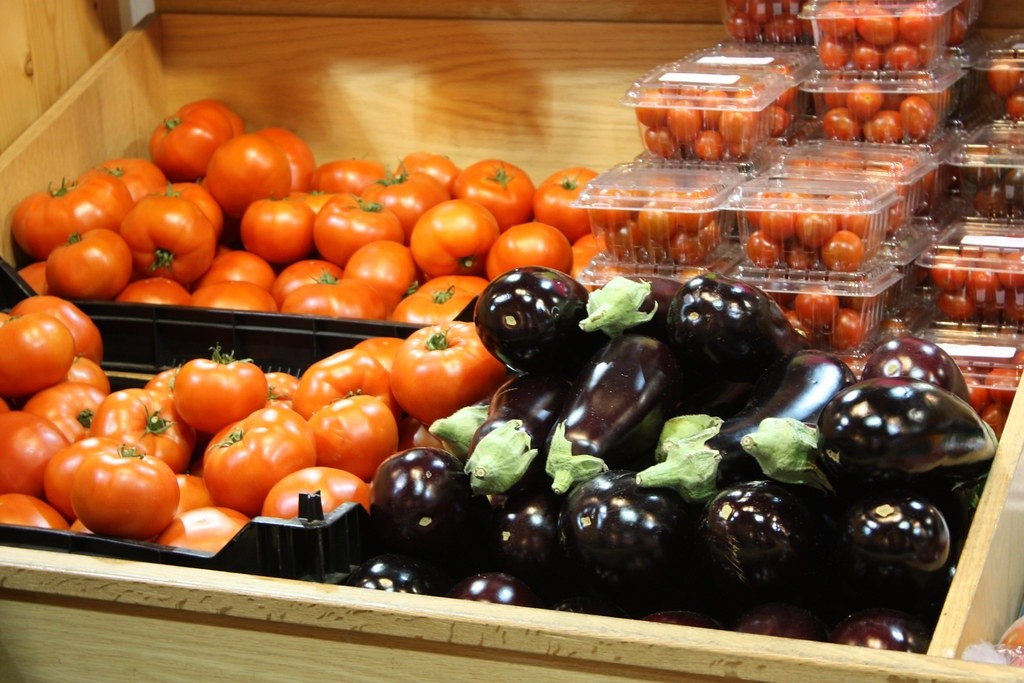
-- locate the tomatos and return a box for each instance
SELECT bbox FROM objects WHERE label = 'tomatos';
[0,0,1024,553]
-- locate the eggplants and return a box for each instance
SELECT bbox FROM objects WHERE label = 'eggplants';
[350,266,998,653]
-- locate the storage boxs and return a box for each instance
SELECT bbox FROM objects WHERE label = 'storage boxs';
[0,259,480,585]
